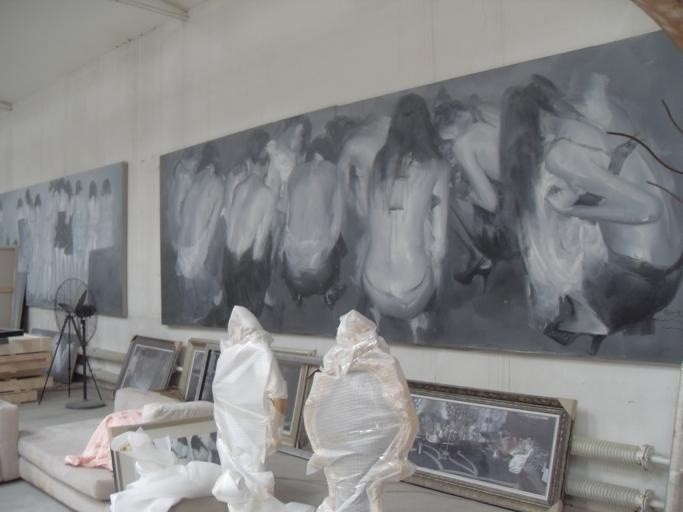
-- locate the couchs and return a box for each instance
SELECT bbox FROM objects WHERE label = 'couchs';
[18,388,214,512]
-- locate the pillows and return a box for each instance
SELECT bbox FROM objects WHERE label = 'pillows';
[65,411,141,467]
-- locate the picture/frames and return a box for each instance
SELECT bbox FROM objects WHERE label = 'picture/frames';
[180,339,323,447]
[111,418,221,492]
[113,336,180,400]
[295,366,575,512]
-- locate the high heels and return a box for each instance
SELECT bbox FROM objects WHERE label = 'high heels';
[263,301,273,310]
[543,320,605,356]
[411,312,430,344]
[454,255,492,293]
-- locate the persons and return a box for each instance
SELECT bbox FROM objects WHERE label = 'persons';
[9,174,113,302]
[170,428,220,466]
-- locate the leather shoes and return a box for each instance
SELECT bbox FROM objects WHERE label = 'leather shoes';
[295,295,302,306]
[325,284,345,310]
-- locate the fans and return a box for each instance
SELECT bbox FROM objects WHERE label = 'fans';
[38,278,105,409]
